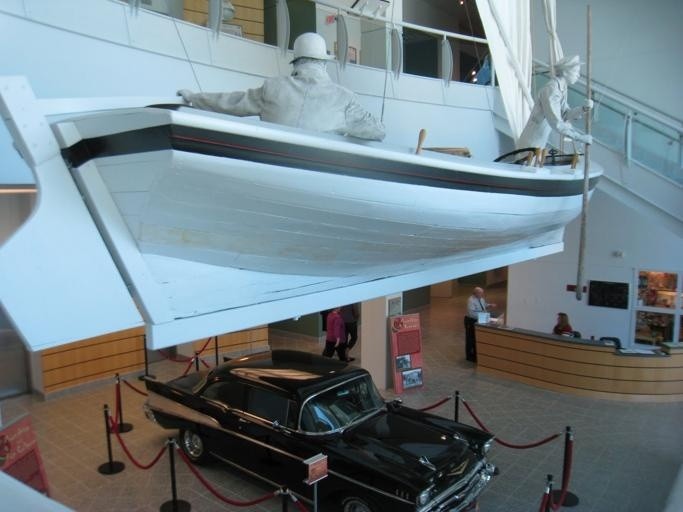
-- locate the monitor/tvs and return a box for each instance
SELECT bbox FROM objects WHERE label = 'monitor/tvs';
[588,280,629,309]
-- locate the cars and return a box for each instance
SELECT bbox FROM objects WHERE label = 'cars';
[130,343,504,512]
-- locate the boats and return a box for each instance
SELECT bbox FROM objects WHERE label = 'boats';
[0,60,608,352]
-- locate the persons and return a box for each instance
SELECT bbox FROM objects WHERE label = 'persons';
[320,307,348,364]
[172,31,387,145]
[463,287,499,363]
[552,312,572,336]
[511,53,594,167]
[339,302,360,362]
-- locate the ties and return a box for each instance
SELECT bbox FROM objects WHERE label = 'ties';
[478,299,483,310]
